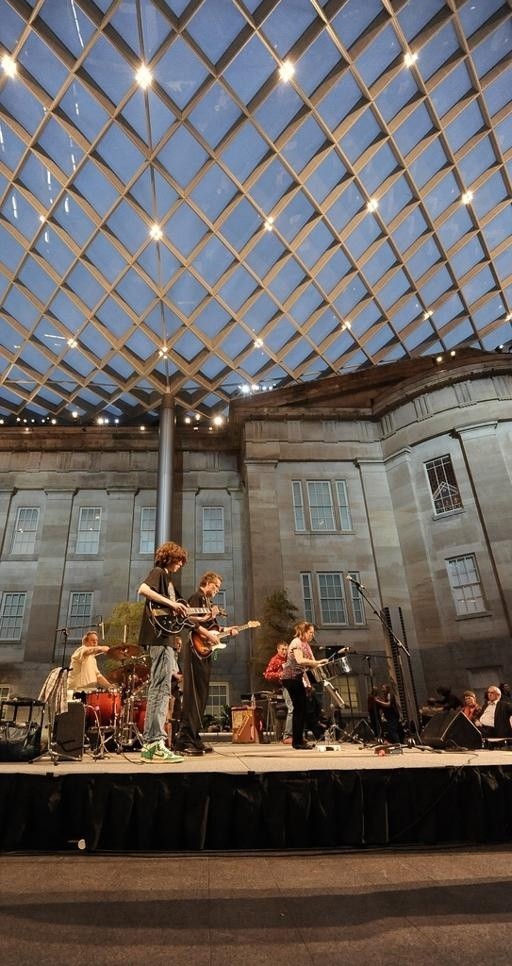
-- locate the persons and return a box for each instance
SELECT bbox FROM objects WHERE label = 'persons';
[281,622,329,750]
[171,635,185,748]
[264,639,309,744]
[375,682,403,743]
[65,629,120,753]
[138,541,220,764]
[419,682,512,746]
[172,570,242,757]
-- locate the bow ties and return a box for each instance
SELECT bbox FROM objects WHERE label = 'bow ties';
[488,701,495,705]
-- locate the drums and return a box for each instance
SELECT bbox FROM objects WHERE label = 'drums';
[86,688,122,725]
[134,701,141,707]
[312,656,352,684]
[139,698,147,730]
[130,707,141,724]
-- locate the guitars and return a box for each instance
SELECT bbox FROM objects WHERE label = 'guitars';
[144,595,229,635]
[188,620,261,659]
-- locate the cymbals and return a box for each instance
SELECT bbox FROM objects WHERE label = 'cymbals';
[108,664,150,683]
[107,644,138,659]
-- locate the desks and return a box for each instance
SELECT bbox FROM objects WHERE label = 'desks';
[1,698,47,728]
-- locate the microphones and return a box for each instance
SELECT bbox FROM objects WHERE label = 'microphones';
[345,575,366,590]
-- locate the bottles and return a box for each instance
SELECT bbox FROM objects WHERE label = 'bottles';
[251,694,257,708]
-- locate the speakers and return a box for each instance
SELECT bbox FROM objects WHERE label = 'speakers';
[419,711,482,749]
[231,706,265,744]
[240,699,272,731]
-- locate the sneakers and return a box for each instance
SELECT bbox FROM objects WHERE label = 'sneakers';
[283,736,312,749]
[174,742,213,756]
[142,738,185,763]
[316,730,324,742]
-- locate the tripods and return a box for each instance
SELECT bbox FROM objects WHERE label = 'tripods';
[312,588,442,754]
[28,622,149,766]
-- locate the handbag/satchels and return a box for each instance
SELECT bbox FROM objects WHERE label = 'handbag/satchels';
[0,719,42,762]
